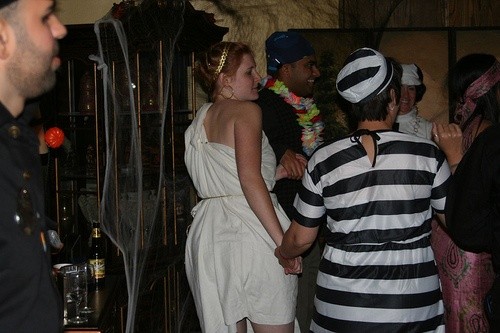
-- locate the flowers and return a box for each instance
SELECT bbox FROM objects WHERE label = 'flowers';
[259,75,326,157]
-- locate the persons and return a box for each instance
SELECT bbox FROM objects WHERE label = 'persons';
[183,40,308,333]
[394,61,436,140]
[0,0,69,333]
[252,32,333,333]
[432,52,500,333]
[275,45,463,333]
[433,124,499,278]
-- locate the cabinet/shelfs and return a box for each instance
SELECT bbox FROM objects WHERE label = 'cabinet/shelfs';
[46,23,197,333]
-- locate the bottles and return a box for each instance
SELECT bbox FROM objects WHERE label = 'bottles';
[88,222,106,291]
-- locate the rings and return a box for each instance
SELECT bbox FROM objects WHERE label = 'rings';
[434,134,439,137]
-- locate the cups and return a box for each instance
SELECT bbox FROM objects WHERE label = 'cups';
[52,263,73,284]
[60,264,88,328]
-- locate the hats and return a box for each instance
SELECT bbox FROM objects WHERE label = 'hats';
[335,47,394,109]
[264,31,314,68]
